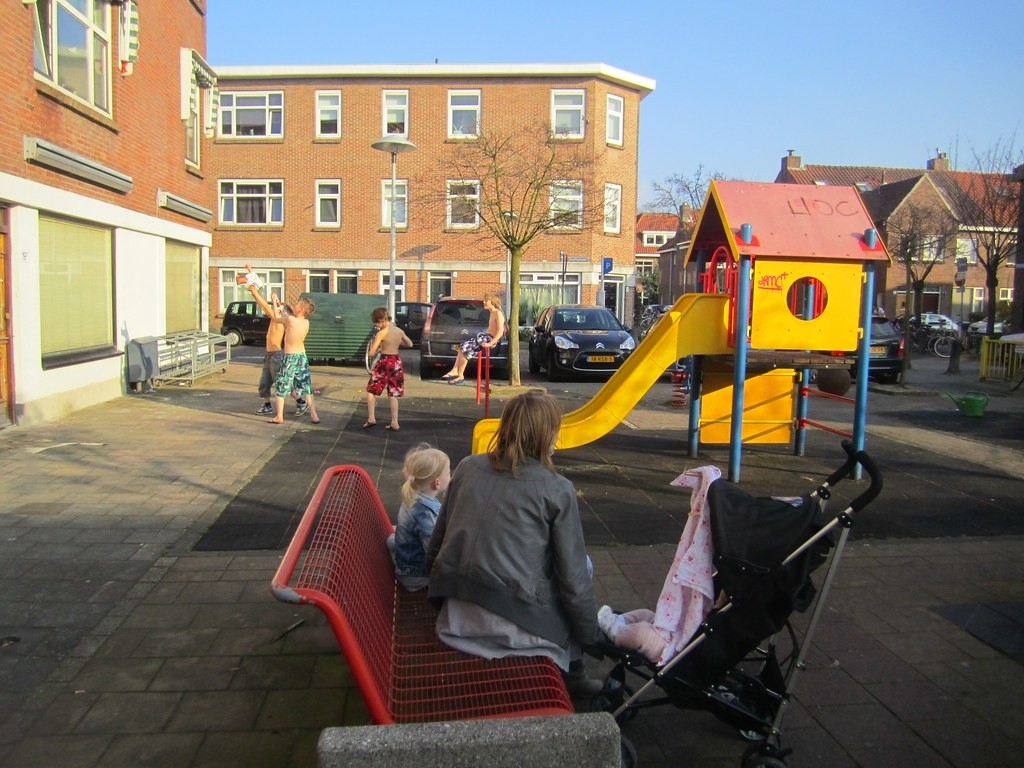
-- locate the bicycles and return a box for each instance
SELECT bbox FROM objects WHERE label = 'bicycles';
[903,318,978,359]
[1010,367,1024,391]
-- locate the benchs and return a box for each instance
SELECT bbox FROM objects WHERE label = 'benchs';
[271,465,573,726]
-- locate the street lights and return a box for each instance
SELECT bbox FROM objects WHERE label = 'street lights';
[502,211,519,325]
[370,131,418,328]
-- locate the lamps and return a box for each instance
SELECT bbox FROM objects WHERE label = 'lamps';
[24,134,134,193]
[157,187,212,223]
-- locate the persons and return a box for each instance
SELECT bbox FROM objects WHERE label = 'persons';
[873,304,885,317]
[427,390,603,696]
[441,294,504,385]
[362,307,413,431]
[598,587,727,662]
[248,286,319,424]
[396,448,450,592]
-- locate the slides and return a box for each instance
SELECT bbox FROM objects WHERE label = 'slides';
[470,292,729,456]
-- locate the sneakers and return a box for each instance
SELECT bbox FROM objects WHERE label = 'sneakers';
[294,398,309,417]
[254,404,274,415]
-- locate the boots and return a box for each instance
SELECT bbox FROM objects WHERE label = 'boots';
[577,668,603,692]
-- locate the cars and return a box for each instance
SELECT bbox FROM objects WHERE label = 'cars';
[895,313,960,337]
[638,303,694,379]
[394,301,434,344]
[528,305,636,382]
[819,315,911,385]
[968,316,1011,340]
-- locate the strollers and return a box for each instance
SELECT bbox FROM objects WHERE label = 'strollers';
[582,439,887,768]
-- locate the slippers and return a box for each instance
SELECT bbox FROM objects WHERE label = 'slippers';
[362,419,376,429]
[267,418,282,424]
[441,373,459,380]
[312,418,320,424]
[385,423,399,432]
[447,379,464,385]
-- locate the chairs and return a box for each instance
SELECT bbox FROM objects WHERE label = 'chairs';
[557,313,563,326]
[585,316,598,324]
[446,308,461,319]
[479,311,489,319]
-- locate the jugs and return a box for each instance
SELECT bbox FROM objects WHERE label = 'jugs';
[945,391,990,417]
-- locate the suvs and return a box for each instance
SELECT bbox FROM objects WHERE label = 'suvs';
[219,300,297,348]
[419,293,510,376]
